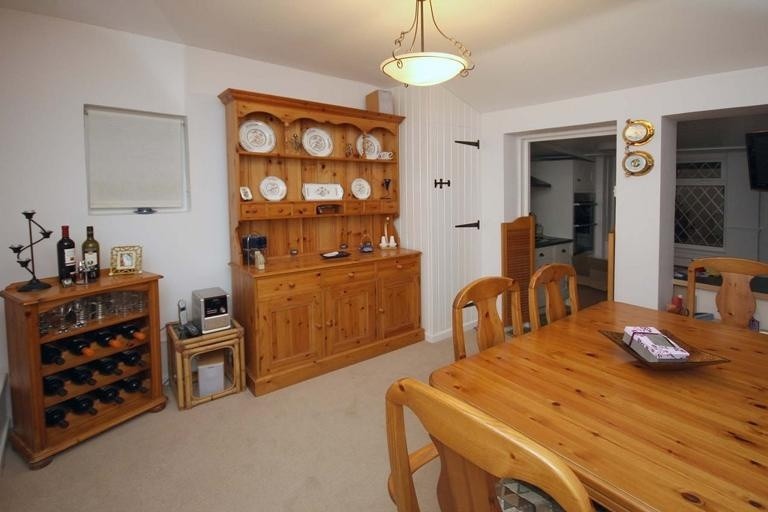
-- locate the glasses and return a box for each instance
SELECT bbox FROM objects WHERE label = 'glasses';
[382,0,475,88]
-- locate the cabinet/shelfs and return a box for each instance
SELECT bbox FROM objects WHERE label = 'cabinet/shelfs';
[94,387,124,405]
[69,397,98,416]
[45,406,68,429]
[41,344,65,365]
[96,330,121,349]
[121,378,148,394]
[119,350,147,367]
[95,358,123,375]
[57,225,76,283]
[82,225,100,278]
[66,338,95,357]
[44,375,68,396]
[67,366,96,386]
[118,324,144,341]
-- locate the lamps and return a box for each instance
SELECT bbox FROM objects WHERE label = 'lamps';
[452,277,523,361]
[527,264,579,329]
[385,377,591,511]
[688,257,768,332]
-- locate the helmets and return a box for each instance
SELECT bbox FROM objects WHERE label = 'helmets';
[239,120,276,153]
[302,183,344,200]
[259,176,287,201]
[351,178,371,199]
[356,133,381,160]
[302,128,334,156]
[596,329,731,371]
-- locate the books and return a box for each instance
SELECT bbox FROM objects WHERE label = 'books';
[576,257,609,294]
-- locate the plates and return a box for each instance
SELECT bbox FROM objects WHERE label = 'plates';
[0,268,169,470]
[533,235,572,317]
[217,88,425,398]
[673,147,768,330]
[532,155,599,256]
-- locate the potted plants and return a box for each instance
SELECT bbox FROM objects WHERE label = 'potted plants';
[622,149,655,177]
[108,246,142,276]
[623,119,654,148]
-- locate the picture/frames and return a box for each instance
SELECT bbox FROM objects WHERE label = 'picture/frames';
[166,317,246,410]
